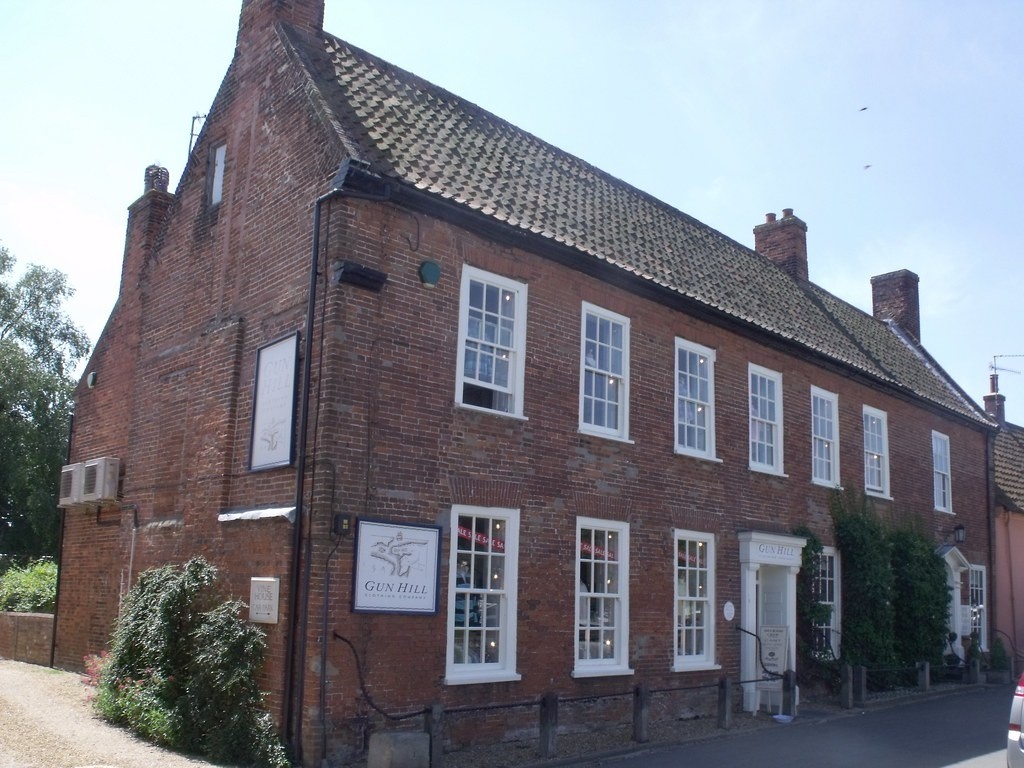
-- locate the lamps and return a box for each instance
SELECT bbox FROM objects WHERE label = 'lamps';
[943,525,965,544]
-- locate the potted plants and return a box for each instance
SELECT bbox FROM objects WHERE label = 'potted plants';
[961,633,1010,685]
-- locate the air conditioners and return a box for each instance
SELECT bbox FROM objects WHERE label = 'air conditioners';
[57,456,120,509]
